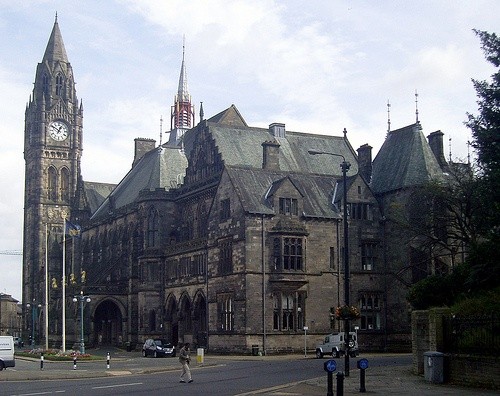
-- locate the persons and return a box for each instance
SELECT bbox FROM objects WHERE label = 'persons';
[179,342,194,384]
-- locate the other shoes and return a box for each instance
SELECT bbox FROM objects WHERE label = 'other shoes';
[188,380,194,383]
[180,381,186,383]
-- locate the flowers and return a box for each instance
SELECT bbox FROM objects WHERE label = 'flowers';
[334,304,361,321]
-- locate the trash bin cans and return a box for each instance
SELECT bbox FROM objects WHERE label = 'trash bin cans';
[252,345,258,356]
[423,352,444,384]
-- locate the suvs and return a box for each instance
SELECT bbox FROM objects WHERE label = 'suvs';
[142,338,177,358]
[315,331,358,359]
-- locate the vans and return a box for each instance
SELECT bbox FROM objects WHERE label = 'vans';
[13,337,20,346]
[0,336,15,371]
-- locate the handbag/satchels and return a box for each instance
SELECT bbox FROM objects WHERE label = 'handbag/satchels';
[186,360,193,368]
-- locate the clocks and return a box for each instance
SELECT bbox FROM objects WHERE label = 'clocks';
[48,120,70,141]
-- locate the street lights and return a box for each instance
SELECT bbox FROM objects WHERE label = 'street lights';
[72,291,92,355]
[26,298,42,349]
[307,148,360,377]
